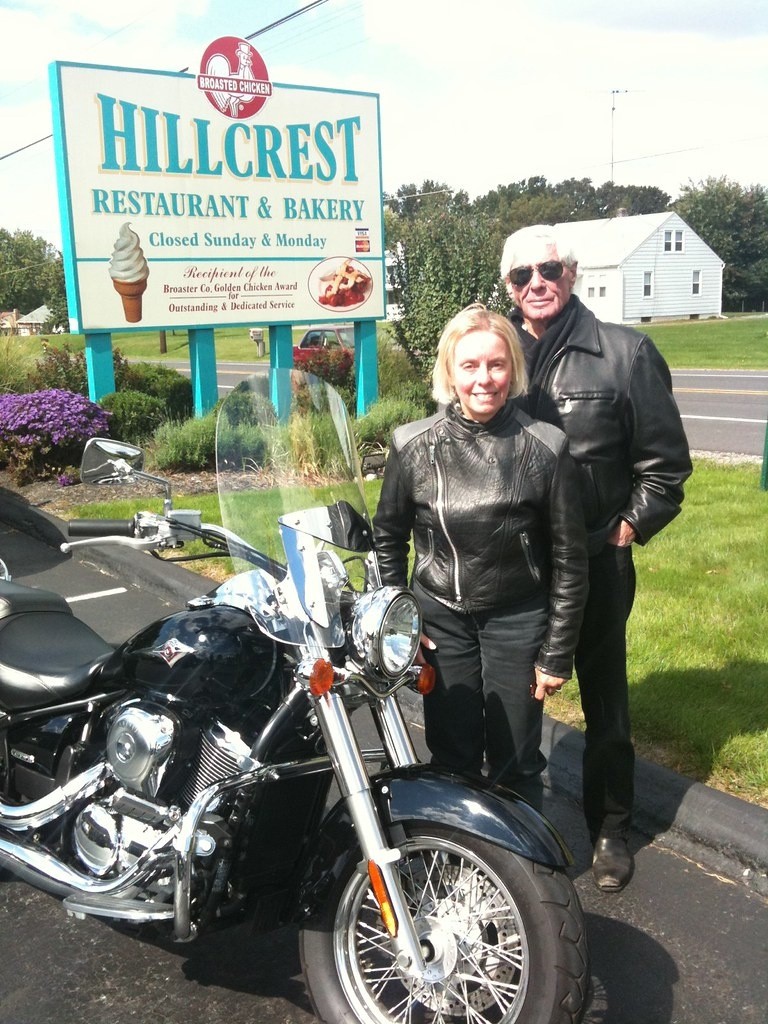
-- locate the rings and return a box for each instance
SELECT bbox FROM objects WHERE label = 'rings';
[555,685,563,693]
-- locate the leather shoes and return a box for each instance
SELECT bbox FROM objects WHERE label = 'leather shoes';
[592,835,634,893]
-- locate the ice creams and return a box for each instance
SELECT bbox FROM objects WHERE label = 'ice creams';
[105,221,153,325]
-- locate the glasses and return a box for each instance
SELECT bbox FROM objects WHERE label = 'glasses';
[508,260,569,285]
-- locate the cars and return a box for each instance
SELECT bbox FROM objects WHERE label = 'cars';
[293,324,356,384]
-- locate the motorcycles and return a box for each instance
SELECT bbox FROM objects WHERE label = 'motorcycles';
[0,368,595,1024]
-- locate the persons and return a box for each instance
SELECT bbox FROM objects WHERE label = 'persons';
[499,226,693,896]
[372,303,593,819]
